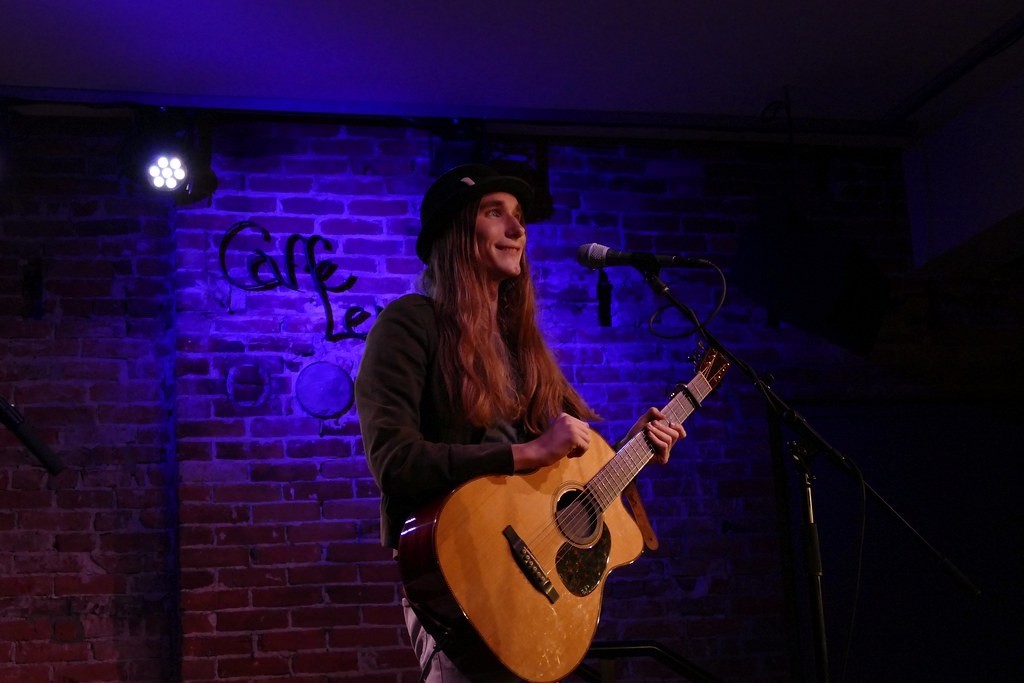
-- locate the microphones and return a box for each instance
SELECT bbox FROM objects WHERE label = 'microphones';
[577,243,711,269]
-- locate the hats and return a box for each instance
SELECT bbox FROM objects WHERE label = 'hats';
[415,159,547,265]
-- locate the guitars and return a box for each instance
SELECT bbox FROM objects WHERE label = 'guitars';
[394,338,733,682]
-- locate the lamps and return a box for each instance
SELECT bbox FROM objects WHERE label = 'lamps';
[126,126,219,203]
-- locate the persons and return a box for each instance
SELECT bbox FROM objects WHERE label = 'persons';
[352,160,689,683]
